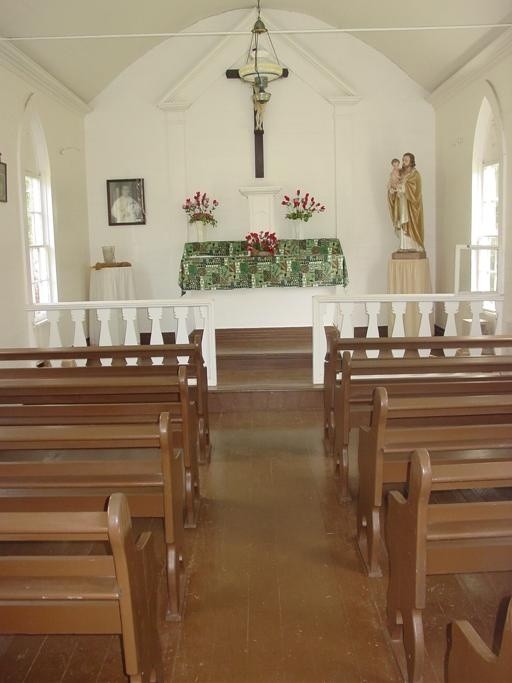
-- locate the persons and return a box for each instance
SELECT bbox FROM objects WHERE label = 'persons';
[387,152,425,252]
[111,185,142,223]
[389,159,404,199]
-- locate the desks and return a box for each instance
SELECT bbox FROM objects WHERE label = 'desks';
[389,252,433,294]
[88,262,140,346]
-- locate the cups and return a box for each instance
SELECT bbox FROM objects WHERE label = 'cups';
[102,244,116,263]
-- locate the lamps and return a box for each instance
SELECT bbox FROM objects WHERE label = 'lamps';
[238,0,283,103]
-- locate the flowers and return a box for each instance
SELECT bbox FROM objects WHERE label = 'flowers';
[182,191,219,228]
[242,231,279,255]
[281,190,326,223]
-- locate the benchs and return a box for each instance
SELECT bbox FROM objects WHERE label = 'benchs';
[0,337,211,683]
[323,325,511,683]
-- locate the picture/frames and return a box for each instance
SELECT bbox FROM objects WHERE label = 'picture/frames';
[0,162,8,203]
[106,177,145,226]
